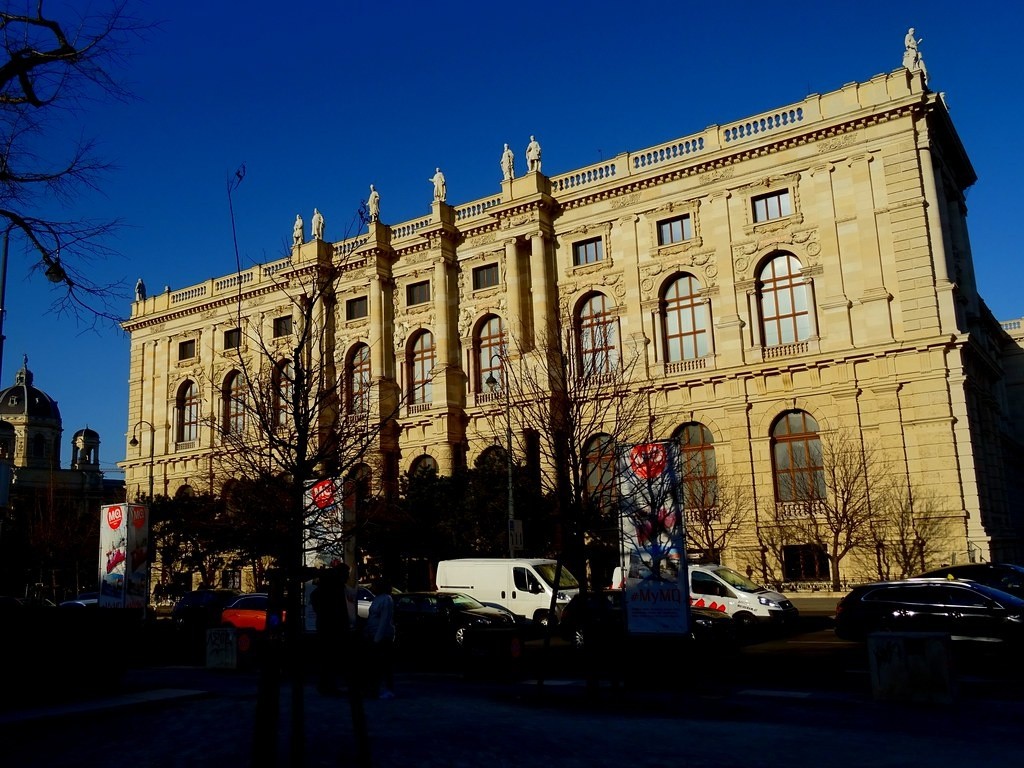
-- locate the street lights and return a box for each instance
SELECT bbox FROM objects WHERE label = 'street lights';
[486,356,517,561]
[131,419,156,622]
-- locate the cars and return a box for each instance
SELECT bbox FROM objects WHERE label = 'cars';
[61,592,100,609]
[912,563,1024,603]
[557,588,737,663]
[218,590,289,633]
[364,591,515,653]
[171,588,244,633]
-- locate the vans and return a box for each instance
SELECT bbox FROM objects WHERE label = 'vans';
[434,555,580,635]
[302,580,377,633]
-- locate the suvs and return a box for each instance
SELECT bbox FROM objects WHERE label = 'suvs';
[608,560,800,639]
[832,572,1024,691]
